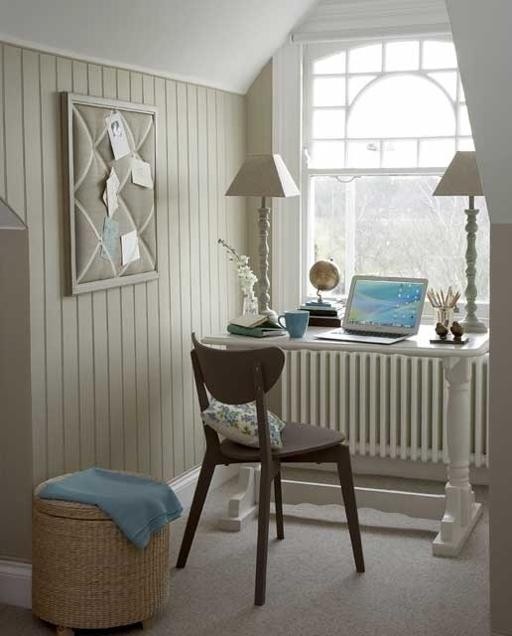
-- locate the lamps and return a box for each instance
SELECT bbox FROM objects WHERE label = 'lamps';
[432,150,489,333]
[223,154,301,327]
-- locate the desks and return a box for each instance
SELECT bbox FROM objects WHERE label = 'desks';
[200,323,489,559]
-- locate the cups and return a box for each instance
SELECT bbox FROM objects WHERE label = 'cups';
[277,309,310,338]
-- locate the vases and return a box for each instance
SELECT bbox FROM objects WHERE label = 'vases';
[243,296,258,314]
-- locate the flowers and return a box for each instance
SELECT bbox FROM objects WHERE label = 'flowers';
[217,237,261,314]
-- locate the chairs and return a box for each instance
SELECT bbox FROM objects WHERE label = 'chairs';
[175,331,365,606]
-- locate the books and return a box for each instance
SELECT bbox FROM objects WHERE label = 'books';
[230,312,268,329]
[226,324,286,338]
[297,301,346,328]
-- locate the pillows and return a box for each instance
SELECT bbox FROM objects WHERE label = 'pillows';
[202,395,286,452]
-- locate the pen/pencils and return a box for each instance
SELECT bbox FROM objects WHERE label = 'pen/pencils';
[426,287,462,307]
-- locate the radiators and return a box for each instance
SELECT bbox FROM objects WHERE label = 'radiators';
[280,351,489,489]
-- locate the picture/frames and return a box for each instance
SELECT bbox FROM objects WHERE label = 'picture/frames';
[57,90,160,296]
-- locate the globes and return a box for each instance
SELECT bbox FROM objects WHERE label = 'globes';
[306,256,341,308]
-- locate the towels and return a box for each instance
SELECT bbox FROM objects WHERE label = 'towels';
[38,465,183,550]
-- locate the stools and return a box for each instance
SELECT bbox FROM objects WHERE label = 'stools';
[30,469,171,636]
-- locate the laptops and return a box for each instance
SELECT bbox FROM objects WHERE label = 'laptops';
[313,275,430,345]
